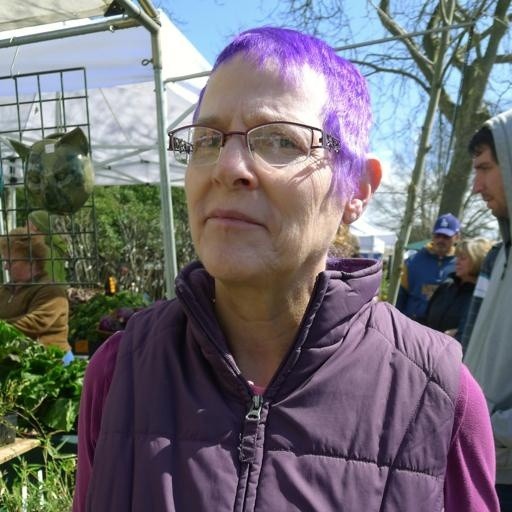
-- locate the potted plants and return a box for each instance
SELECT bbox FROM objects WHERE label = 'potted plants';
[1,370,50,447]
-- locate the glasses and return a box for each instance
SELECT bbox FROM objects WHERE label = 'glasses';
[167,119,345,168]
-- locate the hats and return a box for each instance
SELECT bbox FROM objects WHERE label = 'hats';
[28,210,50,234]
[432,213,460,237]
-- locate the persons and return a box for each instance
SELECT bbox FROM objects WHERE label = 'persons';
[422,235,497,339]
[0,227,76,367]
[464,108,512,511]
[393,213,458,325]
[70,24,501,512]
[24,210,68,293]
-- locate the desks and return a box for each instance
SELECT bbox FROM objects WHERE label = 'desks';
[0,437,46,512]
